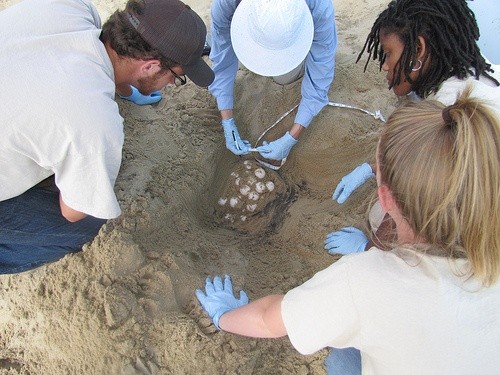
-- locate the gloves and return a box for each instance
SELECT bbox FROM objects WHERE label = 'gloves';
[332,162,375,205]
[195,274,249,330]
[256,131,298,160]
[119,85,161,105]
[222,117,253,155]
[324,227,370,256]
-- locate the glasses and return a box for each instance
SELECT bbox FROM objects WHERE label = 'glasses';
[168,67,187,87]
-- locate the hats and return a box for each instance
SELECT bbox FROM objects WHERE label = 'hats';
[230,0,314,77]
[126,0,216,87]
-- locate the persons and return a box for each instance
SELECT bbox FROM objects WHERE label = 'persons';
[324,0,500,254]
[208,0,337,160]
[0,0,215,274]
[195,84,500,375]
